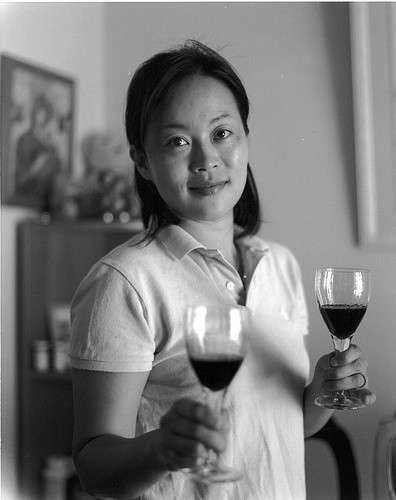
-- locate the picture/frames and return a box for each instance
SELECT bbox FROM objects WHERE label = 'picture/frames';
[1,53,77,214]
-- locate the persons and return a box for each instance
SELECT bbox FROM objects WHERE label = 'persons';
[67,40,377,500]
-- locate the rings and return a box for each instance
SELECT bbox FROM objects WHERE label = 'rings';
[359,371,366,388]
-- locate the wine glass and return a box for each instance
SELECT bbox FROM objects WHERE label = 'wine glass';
[179,304,249,484]
[314,267,371,411]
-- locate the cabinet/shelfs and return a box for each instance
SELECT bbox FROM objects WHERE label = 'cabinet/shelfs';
[15,220,143,498]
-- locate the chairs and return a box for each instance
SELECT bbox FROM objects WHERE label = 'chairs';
[304,414,361,500]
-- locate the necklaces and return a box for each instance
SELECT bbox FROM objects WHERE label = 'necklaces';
[234,254,240,269]
